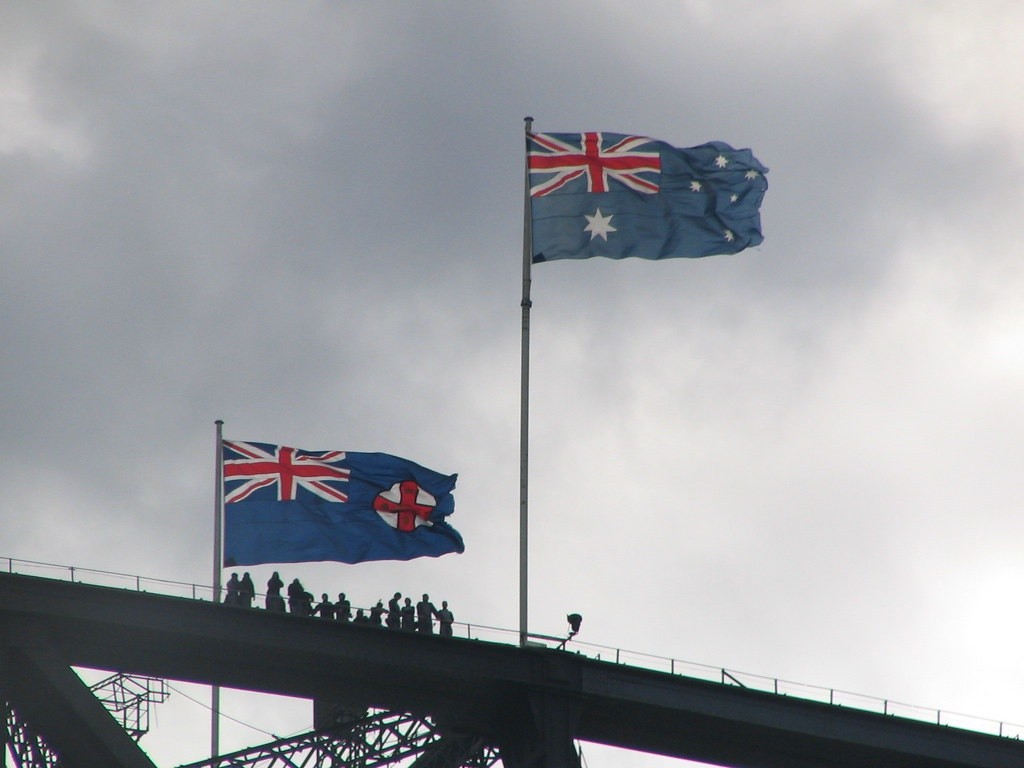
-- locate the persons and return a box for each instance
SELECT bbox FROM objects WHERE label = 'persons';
[437,601,454,636]
[227,572,255,607]
[417,594,438,634]
[354,609,368,624]
[267,572,283,596]
[370,603,388,625]
[288,579,314,616]
[314,593,351,621]
[401,598,415,632]
[389,592,401,628]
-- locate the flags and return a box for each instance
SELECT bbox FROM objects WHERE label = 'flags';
[528,130,769,261]
[222,439,466,568]
